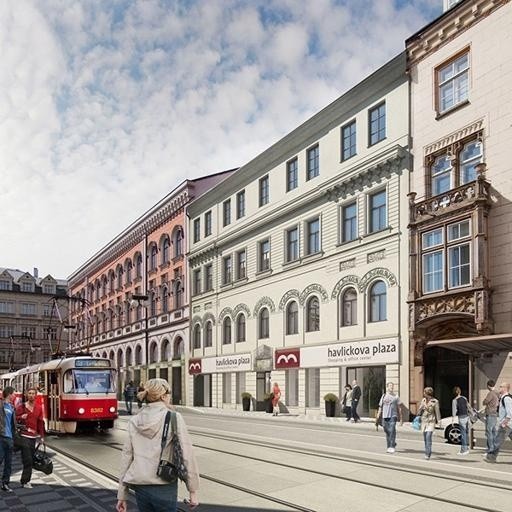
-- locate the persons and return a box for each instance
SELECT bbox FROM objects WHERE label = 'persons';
[14,387,46,492]
[122,379,136,416]
[375,381,404,454]
[349,378,362,424]
[115,377,202,511]
[481,382,512,464]
[339,383,354,422]
[271,382,282,417]
[450,386,475,456]
[0,386,18,494]
[415,386,443,461]
[481,379,501,454]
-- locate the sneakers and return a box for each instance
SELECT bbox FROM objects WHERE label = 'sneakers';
[386,448,395,453]
[20,481,33,488]
[2,483,14,495]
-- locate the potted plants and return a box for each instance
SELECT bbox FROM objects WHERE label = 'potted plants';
[263,392,274,413]
[241,392,251,410]
[324,393,338,417]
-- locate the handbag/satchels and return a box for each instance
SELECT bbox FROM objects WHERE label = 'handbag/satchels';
[412,416,421,430]
[32,443,52,474]
[13,436,26,452]
[377,395,384,426]
[157,433,186,479]
